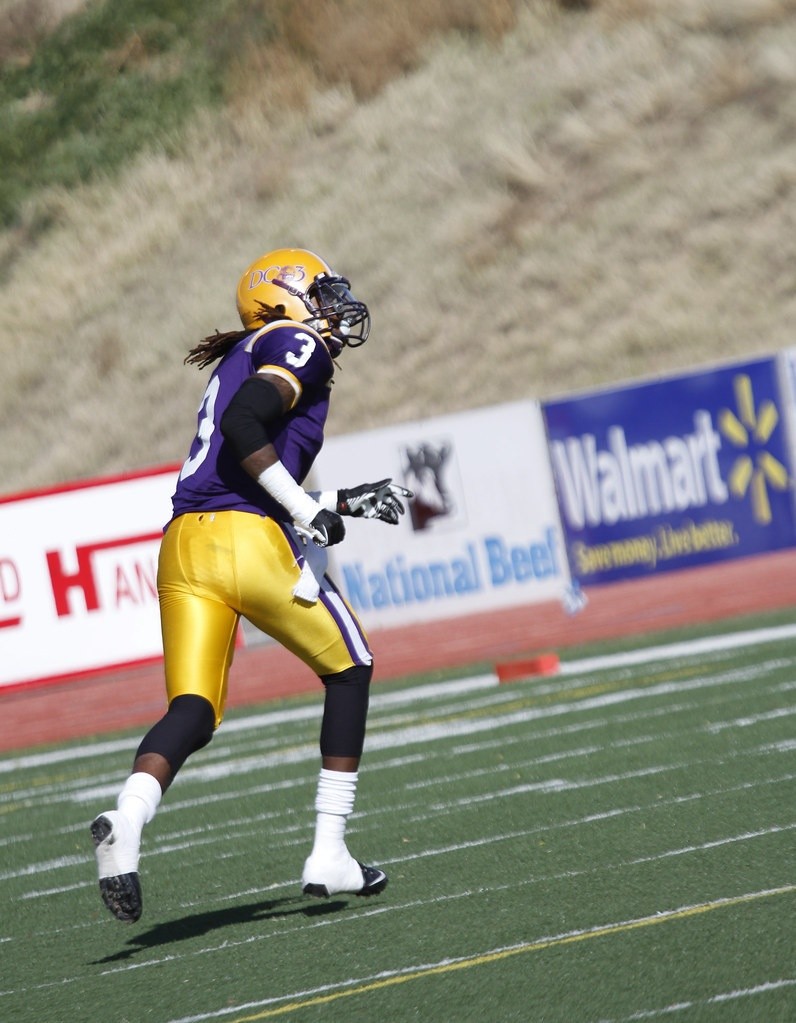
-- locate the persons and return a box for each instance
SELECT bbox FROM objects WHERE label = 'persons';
[89,248,413,924]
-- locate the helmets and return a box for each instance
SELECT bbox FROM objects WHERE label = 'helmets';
[236,248,372,358]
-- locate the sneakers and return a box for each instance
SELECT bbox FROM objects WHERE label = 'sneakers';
[302,856,388,900]
[89,810,143,925]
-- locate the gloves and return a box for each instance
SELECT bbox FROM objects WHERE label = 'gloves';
[335,477,415,526]
[290,498,347,549]
